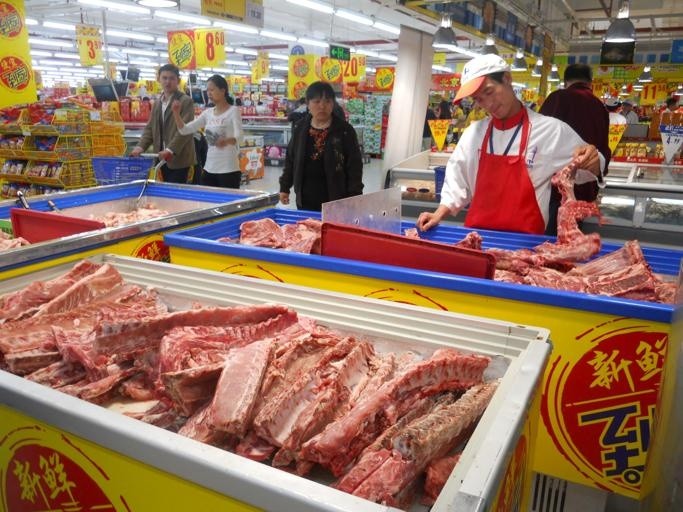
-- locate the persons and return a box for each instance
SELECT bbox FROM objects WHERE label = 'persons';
[126,62,195,187]
[276,80,363,212]
[138,87,306,136]
[169,74,244,192]
[423,98,474,153]
[599,93,680,127]
[528,61,613,240]
[411,51,608,242]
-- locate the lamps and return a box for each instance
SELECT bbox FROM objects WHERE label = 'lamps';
[431,62,455,75]
[600,8,638,44]
[480,33,501,58]
[510,81,526,88]
[511,50,528,72]
[546,64,561,82]
[26,3,415,86]
[616,64,658,97]
[430,11,458,48]
[530,59,544,78]
[450,45,479,62]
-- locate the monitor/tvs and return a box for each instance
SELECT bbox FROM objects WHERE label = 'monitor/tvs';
[599,42,636,67]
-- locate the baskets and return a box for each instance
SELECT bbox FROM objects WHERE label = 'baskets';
[90,154,153,186]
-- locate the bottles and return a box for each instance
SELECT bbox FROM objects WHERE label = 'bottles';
[449,128,458,150]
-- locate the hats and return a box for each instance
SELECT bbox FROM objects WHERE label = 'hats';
[451,53,511,105]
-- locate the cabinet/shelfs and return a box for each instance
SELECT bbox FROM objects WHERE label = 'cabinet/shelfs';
[0,100,292,200]
[239,146,265,186]
[389,151,683,246]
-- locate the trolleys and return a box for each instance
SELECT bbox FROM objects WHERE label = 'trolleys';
[91,153,167,186]
